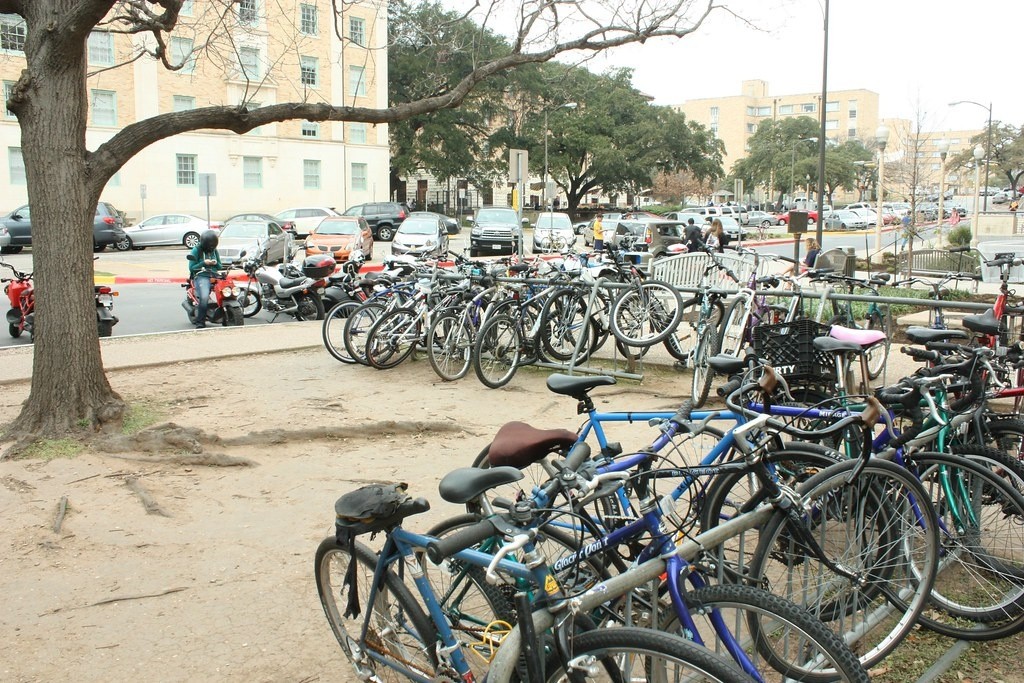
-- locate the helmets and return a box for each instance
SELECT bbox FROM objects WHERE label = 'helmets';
[200,230,218,252]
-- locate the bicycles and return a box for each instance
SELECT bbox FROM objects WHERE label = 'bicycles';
[313,237,1024,682]
[540,229,568,255]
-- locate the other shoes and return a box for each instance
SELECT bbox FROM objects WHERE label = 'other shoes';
[196,320,205,328]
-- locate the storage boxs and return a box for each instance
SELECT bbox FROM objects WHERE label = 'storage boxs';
[303,255,337,278]
[667,243,690,258]
[754,321,838,385]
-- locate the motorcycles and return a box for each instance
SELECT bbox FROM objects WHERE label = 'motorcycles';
[237,241,440,323]
[181,250,247,328]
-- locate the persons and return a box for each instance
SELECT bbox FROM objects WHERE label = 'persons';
[665,217,726,254]
[592,213,608,251]
[188,230,223,329]
[405,198,437,212]
[781,238,829,291]
[901,212,915,251]
[950,208,960,228]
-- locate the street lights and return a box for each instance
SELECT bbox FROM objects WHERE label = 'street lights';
[876,119,890,266]
[791,136,819,215]
[546,102,577,212]
[949,101,992,213]
[973,143,986,254]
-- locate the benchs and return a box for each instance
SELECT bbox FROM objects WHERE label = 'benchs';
[802,249,847,291]
[899,248,983,280]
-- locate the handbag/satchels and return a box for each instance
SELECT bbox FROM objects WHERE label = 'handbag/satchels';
[706,231,720,248]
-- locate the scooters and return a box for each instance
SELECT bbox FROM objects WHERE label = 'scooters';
[0,255,120,341]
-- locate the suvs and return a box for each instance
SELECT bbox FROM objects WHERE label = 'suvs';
[342,202,410,242]
[0,200,126,253]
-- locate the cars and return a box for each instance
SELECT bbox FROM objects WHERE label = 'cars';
[0,223,11,252]
[793,185,967,230]
[216,220,297,269]
[613,220,688,261]
[979,186,1024,204]
[225,213,297,238]
[109,213,225,251]
[409,212,461,235]
[776,209,818,227]
[305,215,374,263]
[391,216,449,258]
[573,203,779,248]
[531,211,577,254]
[274,206,342,237]
[466,205,530,257]
